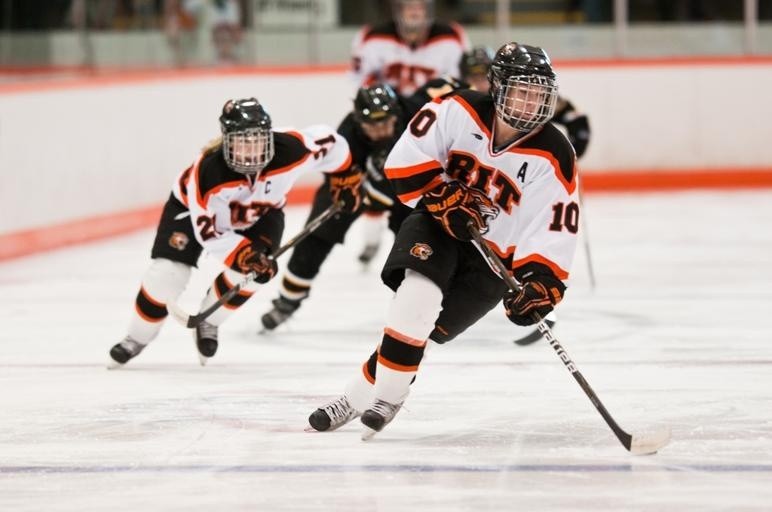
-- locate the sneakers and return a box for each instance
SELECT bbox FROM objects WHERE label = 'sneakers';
[262,308,290,329]
[361,401,402,430]
[308,395,356,432]
[196,319,218,356]
[110,336,145,364]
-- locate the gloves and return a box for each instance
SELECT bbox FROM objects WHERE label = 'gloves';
[502,271,553,326]
[422,179,489,241]
[237,244,279,285]
[329,167,363,211]
[563,108,591,158]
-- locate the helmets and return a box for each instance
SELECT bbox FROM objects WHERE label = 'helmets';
[353,84,398,138]
[487,43,557,133]
[219,97,274,175]
[459,48,489,85]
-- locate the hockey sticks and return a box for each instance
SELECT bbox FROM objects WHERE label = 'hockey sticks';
[165,199,346,328]
[466,218,672,455]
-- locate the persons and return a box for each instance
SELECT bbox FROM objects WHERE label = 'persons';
[110,97,365,364]
[193,22,255,64]
[262,76,476,329]
[348,0,474,264]
[458,47,590,160]
[165,1,239,60]
[309,43,580,432]
